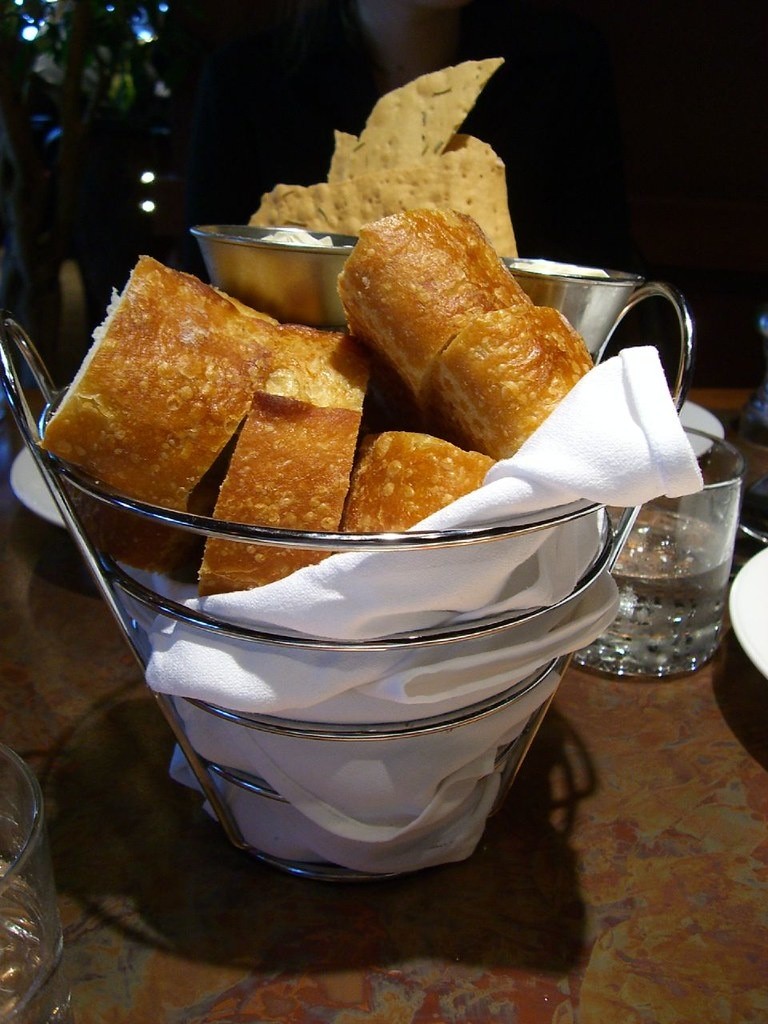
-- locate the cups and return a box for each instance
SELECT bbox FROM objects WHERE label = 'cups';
[0,742,74,1024]
[575,429,743,676]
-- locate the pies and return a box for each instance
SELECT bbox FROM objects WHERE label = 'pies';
[248,55,520,259]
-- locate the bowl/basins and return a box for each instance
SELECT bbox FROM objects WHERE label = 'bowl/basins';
[190,224,643,351]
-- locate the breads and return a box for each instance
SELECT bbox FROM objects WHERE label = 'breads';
[37,208,591,597]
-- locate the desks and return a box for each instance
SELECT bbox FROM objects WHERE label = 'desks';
[0,384,768,1024]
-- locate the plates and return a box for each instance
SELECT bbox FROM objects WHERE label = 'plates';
[679,400,725,459]
[728,546,768,680]
[11,448,66,528]
[737,475,768,543]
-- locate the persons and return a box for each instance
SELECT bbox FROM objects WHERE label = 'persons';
[103,0,648,349]
